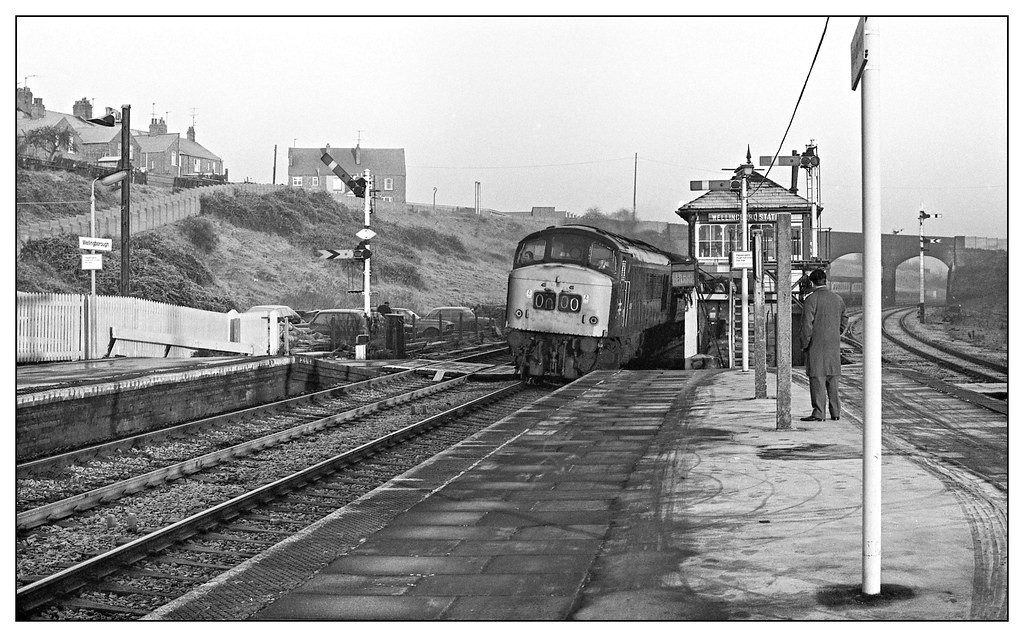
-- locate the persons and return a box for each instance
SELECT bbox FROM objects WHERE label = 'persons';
[522,252,534,263]
[797,269,848,422]
[377,302,391,315]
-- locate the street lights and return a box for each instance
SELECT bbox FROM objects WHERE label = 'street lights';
[90,169,131,359]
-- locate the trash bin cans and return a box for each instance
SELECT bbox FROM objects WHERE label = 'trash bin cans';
[384,313,406,358]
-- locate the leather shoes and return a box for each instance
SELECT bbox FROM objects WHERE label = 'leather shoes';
[831,417,840,420]
[800,416,825,421]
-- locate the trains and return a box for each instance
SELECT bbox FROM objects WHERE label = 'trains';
[504,224,690,380]
